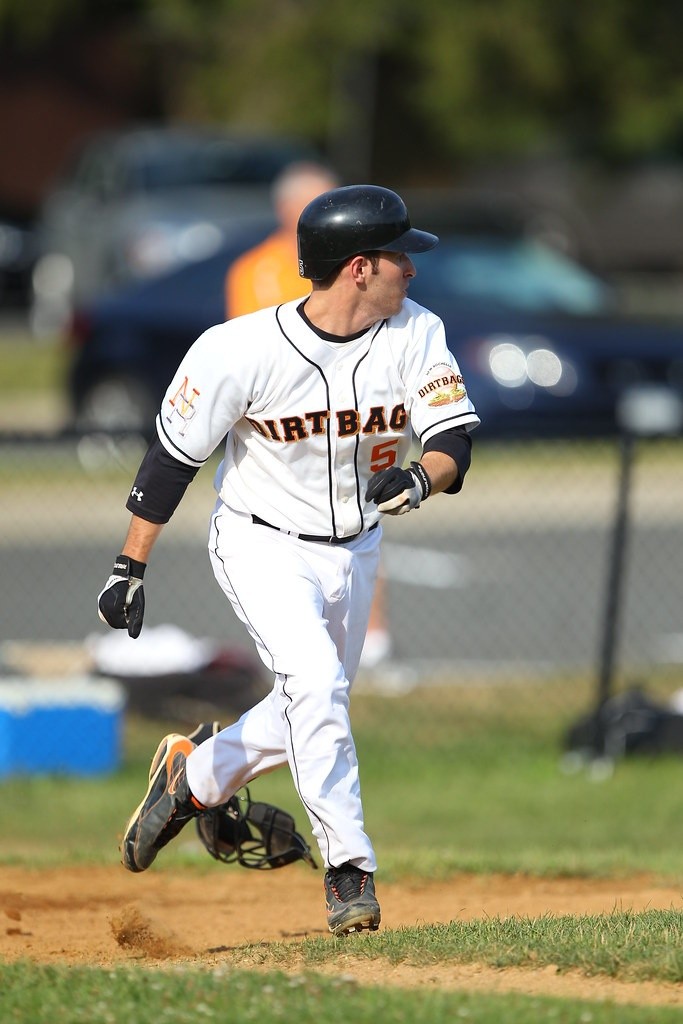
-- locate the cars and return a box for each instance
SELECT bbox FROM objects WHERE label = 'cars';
[68,233,683,448]
[39,131,322,327]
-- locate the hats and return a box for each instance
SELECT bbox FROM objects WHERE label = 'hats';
[297,184,440,281]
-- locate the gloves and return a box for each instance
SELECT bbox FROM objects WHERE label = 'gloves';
[98,555,147,639]
[365,462,431,516]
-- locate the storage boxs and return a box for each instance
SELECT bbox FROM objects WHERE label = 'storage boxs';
[0,674,128,779]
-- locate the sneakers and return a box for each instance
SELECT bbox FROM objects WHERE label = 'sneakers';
[322,865,381,936]
[119,733,206,872]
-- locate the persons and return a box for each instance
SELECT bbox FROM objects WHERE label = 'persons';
[223,161,418,699]
[96,185,483,937]
